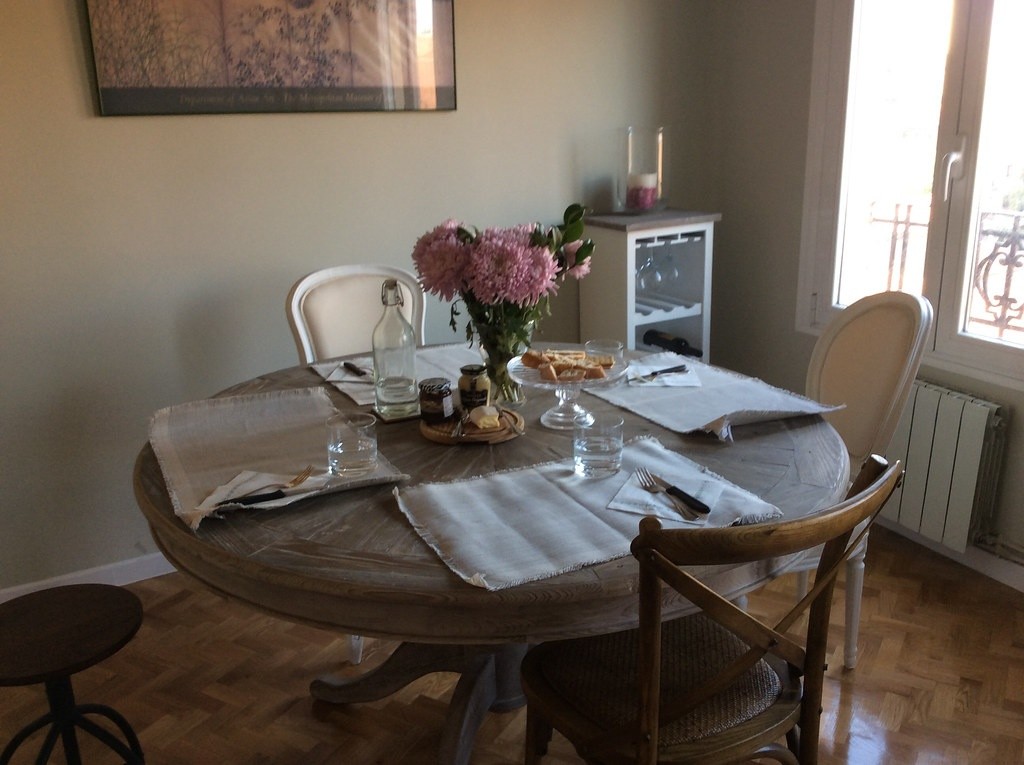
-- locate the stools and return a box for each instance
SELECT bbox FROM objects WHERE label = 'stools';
[0,584,147,765]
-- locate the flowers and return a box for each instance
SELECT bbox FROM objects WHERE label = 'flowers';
[411,204,597,405]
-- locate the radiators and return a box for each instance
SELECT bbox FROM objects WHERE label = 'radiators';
[874,377,1006,553]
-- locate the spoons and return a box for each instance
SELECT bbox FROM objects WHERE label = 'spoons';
[448,403,472,438]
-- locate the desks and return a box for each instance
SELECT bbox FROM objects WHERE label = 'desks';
[132,338,850,764]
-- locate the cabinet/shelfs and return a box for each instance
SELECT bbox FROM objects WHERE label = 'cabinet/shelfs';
[577,218,714,364]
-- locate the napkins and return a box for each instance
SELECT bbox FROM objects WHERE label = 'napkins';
[606,469,725,525]
[625,364,700,387]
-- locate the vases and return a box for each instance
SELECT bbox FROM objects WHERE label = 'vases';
[469,317,535,410]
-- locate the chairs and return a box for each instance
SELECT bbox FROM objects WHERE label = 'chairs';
[284,266,426,667]
[726,288,934,670]
[517,453,906,765]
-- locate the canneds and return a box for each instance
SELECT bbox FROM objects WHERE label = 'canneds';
[419,378,454,422]
[458,364,491,411]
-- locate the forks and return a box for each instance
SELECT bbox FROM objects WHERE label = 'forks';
[237,465,315,500]
[639,369,688,384]
[634,465,701,522]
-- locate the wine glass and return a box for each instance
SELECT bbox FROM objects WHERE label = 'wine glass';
[660,237,685,287]
[640,244,666,294]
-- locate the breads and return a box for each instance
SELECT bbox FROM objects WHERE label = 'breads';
[469,405,499,428]
[523,349,613,382]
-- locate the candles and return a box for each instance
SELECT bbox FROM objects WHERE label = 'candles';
[627,125,632,177]
[657,126,664,200]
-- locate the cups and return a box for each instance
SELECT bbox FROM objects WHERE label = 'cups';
[583,339,624,364]
[325,414,380,475]
[573,411,625,478]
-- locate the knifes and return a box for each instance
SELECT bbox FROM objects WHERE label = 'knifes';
[644,472,712,515]
[627,362,685,386]
[493,403,526,437]
[215,485,329,505]
[341,361,375,384]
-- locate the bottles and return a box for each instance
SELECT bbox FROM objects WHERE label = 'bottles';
[613,123,662,213]
[372,279,420,416]
